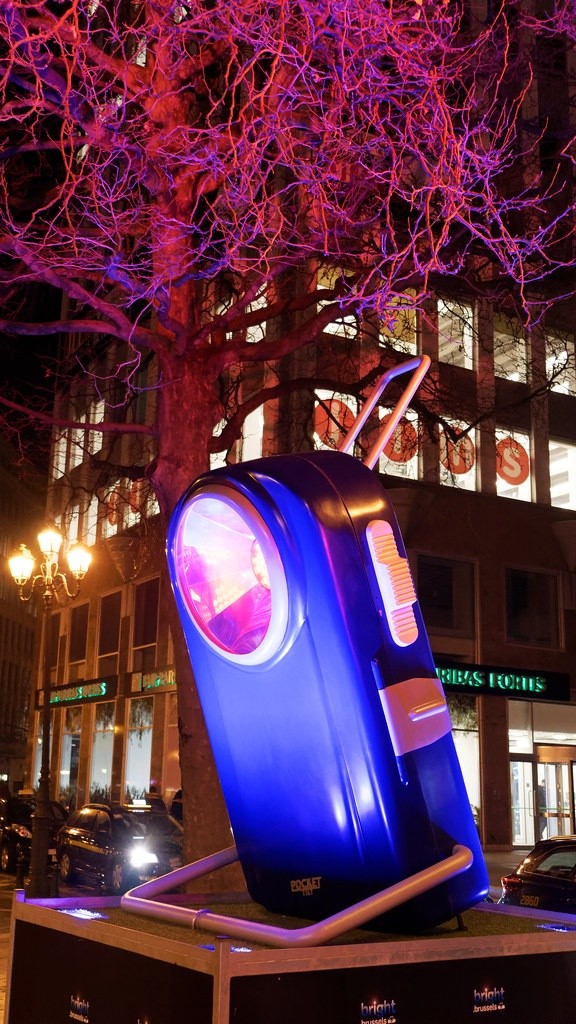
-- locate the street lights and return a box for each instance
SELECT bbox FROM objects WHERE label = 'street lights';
[8,519,92,900]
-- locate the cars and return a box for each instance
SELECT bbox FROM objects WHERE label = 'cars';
[498,835,575,915]
[0,796,69,874]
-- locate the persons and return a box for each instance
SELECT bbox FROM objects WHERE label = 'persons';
[145,786,168,818]
[170,790,184,828]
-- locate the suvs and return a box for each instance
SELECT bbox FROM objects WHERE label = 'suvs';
[57,803,185,897]
